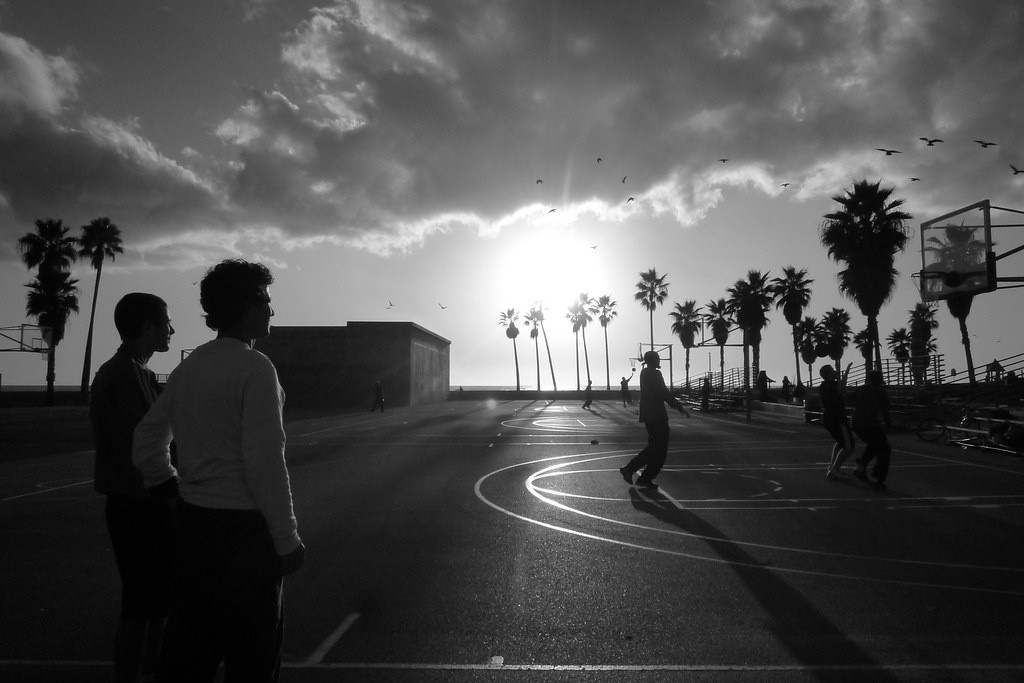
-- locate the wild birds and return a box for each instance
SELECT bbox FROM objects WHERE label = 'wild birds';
[549,209,556,214]
[596,157,602,164]
[1008,164,1024,175]
[875,147,903,156]
[918,137,943,147]
[536,179,543,184]
[974,140,997,148]
[591,245,598,250]
[718,158,730,164]
[909,177,921,182]
[437,302,448,309]
[386,300,394,309]
[622,176,627,184]
[781,183,790,187]
[626,197,635,203]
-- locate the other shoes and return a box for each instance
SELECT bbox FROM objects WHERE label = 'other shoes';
[869,480,887,494]
[827,467,849,483]
[637,477,659,490]
[851,467,869,483]
[620,465,634,485]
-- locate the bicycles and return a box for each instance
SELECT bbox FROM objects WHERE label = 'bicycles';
[915,394,994,444]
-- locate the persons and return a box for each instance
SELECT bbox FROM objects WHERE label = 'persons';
[782,376,791,394]
[132,261,305,683]
[621,377,632,408]
[793,381,806,406]
[620,351,690,489]
[843,369,893,482]
[370,379,384,414]
[818,363,856,480]
[88,293,182,682]
[701,378,710,413]
[582,382,592,409]
[756,371,772,403]
